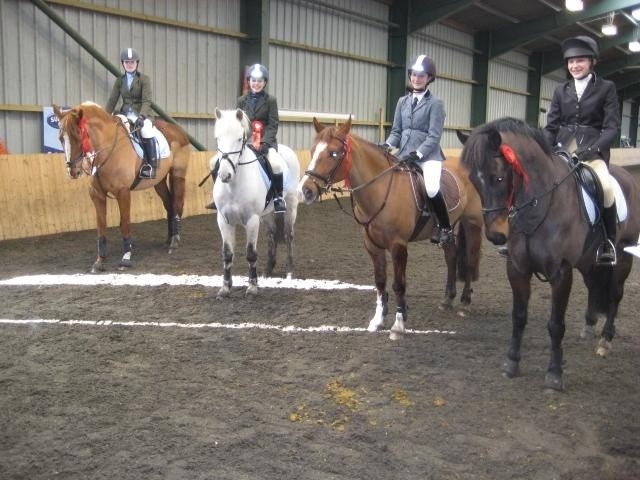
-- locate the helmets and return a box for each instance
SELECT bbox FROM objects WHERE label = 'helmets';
[407,53,437,84]
[246,63,270,82]
[560,34,601,62]
[120,47,141,62]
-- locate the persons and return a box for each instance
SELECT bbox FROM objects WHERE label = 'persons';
[499,32,625,268]
[105,48,159,180]
[377,54,456,245]
[206,62,288,215]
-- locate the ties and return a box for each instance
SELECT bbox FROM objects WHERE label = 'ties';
[410,96,419,112]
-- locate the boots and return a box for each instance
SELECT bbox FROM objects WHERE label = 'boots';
[139,136,158,179]
[269,171,287,214]
[425,188,455,246]
[495,244,510,258]
[204,167,219,210]
[593,195,620,267]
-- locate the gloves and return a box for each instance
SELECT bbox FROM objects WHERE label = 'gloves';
[401,151,420,166]
[571,145,599,163]
[258,142,270,157]
[133,115,145,130]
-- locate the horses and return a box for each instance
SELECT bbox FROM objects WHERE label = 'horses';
[296,113,484,342]
[50,100,190,275]
[454,116,640,401]
[211,107,302,301]
